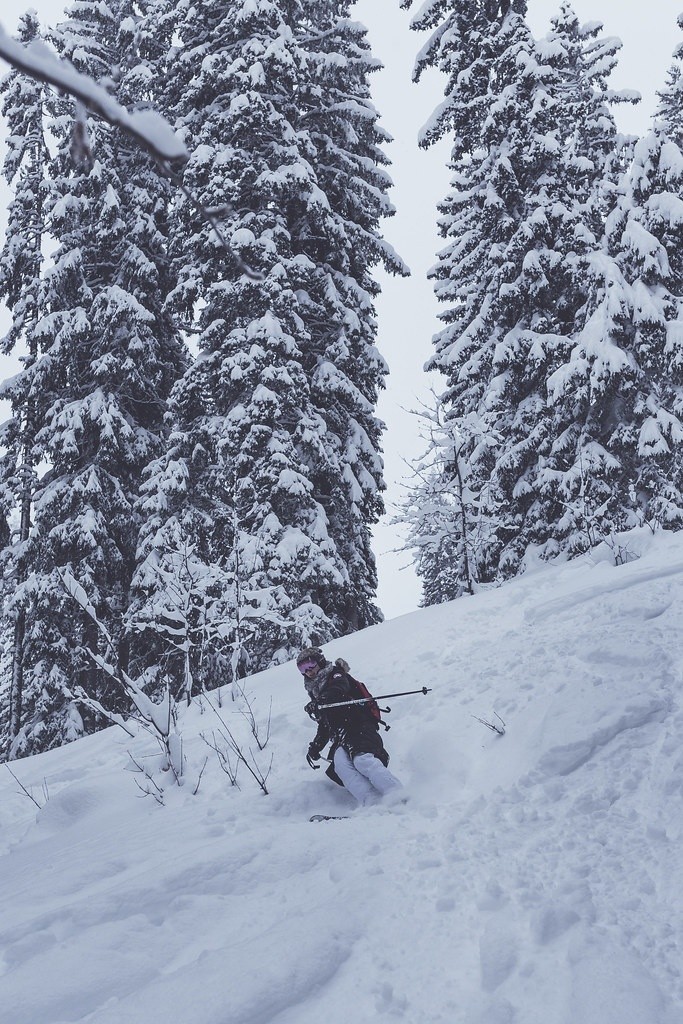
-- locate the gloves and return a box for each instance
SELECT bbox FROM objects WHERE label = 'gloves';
[308,742,321,760]
[304,702,319,714]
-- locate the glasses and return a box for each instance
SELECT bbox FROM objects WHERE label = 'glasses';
[298,659,318,674]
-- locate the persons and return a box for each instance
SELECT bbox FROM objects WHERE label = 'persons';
[296,647,401,806]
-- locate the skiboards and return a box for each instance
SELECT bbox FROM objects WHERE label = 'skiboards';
[308,814,352,822]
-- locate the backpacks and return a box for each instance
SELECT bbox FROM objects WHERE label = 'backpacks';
[330,669,381,720]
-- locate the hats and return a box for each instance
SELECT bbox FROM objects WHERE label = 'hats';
[297,647,326,675]
